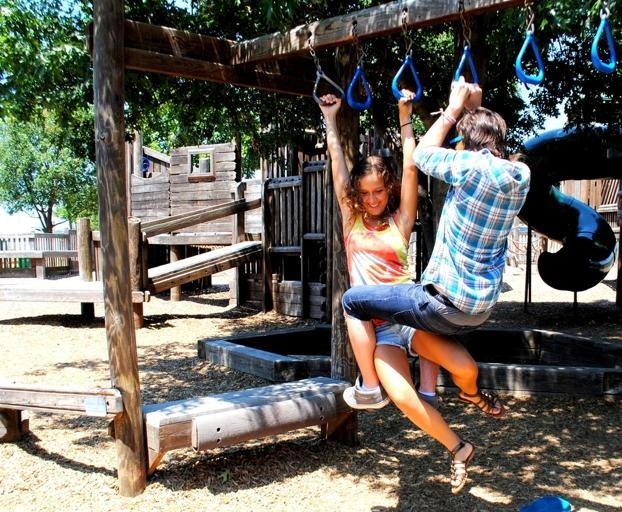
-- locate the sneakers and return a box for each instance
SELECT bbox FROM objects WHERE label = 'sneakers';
[417,391,439,409]
[343,375,391,409]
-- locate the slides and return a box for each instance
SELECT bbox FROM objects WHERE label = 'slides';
[513,122,620,291]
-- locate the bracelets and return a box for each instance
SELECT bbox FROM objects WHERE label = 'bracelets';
[400,121,412,128]
[430,107,457,125]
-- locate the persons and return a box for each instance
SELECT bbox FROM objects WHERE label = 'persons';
[340,76,532,412]
[319,89,507,494]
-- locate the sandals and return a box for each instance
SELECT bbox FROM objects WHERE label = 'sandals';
[450,441,476,494]
[457,388,505,417]
